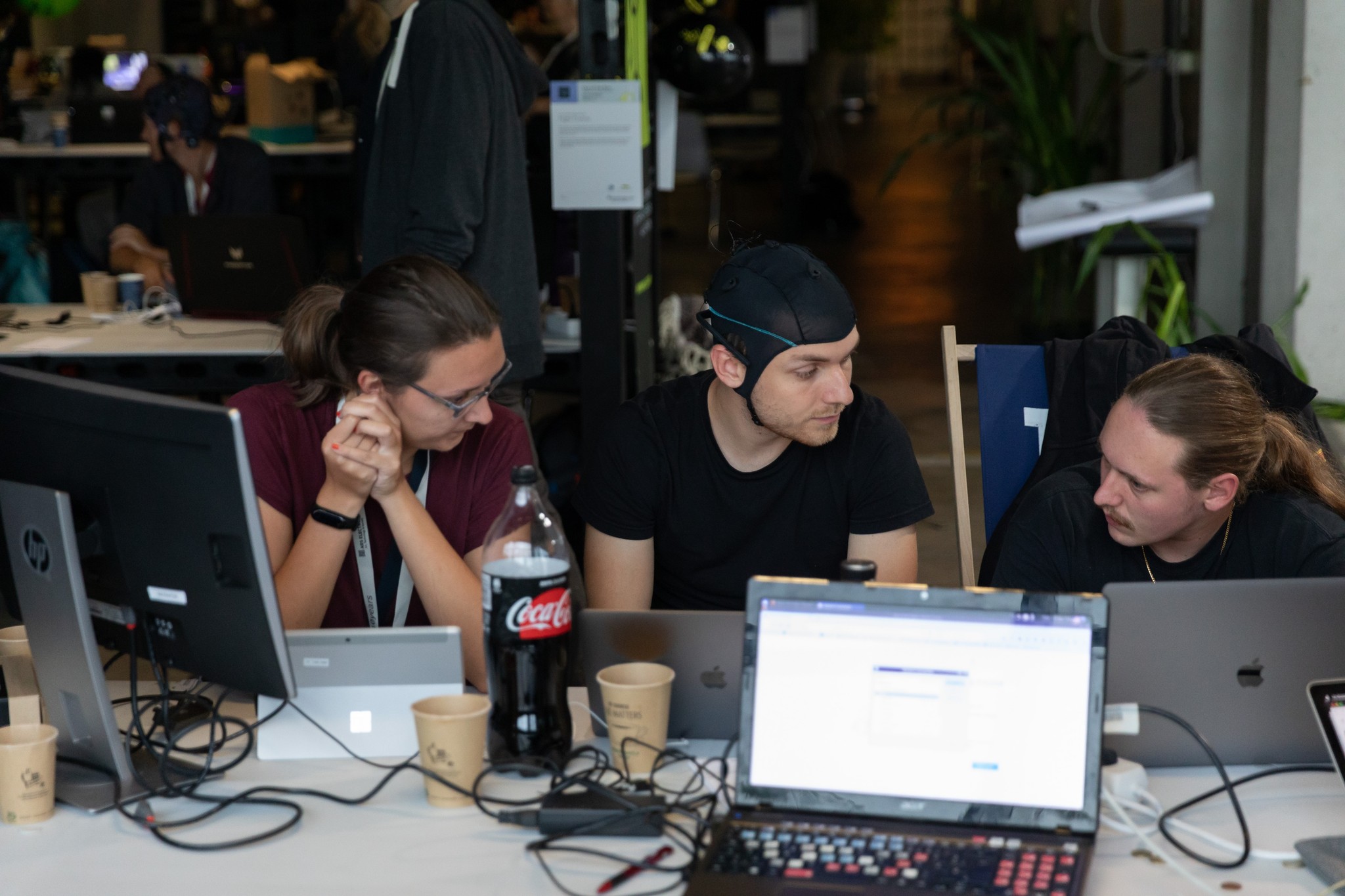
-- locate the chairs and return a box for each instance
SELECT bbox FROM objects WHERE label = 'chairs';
[942,323,1278,591]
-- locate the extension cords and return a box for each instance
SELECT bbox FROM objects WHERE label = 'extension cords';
[1102,758,1148,807]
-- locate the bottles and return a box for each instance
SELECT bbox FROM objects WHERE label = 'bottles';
[478,466,572,777]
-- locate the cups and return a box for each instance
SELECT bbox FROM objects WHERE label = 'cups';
[0,626,39,697]
[81,271,116,313]
[412,695,492,807]
[594,662,676,775]
[116,272,146,312]
[0,721,60,823]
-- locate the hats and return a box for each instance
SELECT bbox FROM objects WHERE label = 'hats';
[699,237,855,398]
[144,73,214,137]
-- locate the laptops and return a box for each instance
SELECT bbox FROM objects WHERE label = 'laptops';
[682,575,1111,896]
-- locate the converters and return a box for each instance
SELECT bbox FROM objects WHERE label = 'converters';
[540,795,667,838]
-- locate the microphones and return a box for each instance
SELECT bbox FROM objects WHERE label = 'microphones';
[697,315,752,367]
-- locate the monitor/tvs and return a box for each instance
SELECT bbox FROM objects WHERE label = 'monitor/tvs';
[576,609,745,740]
[0,365,298,815]
[102,49,153,94]
[1102,579,1344,770]
[1305,678,1345,782]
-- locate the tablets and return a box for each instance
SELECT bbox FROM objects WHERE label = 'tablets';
[257,625,467,762]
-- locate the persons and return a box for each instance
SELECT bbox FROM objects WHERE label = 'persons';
[584,239,934,613]
[355,1,586,613]
[974,356,1345,593]
[217,248,540,630]
[109,76,272,312]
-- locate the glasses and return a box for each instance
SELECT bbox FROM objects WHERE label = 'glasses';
[407,357,513,421]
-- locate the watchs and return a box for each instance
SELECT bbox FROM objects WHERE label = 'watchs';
[308,497,362,532]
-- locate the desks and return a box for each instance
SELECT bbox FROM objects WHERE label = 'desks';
[0,137,354,157]
[0,635,1343,896]
[0,299,296,359]
